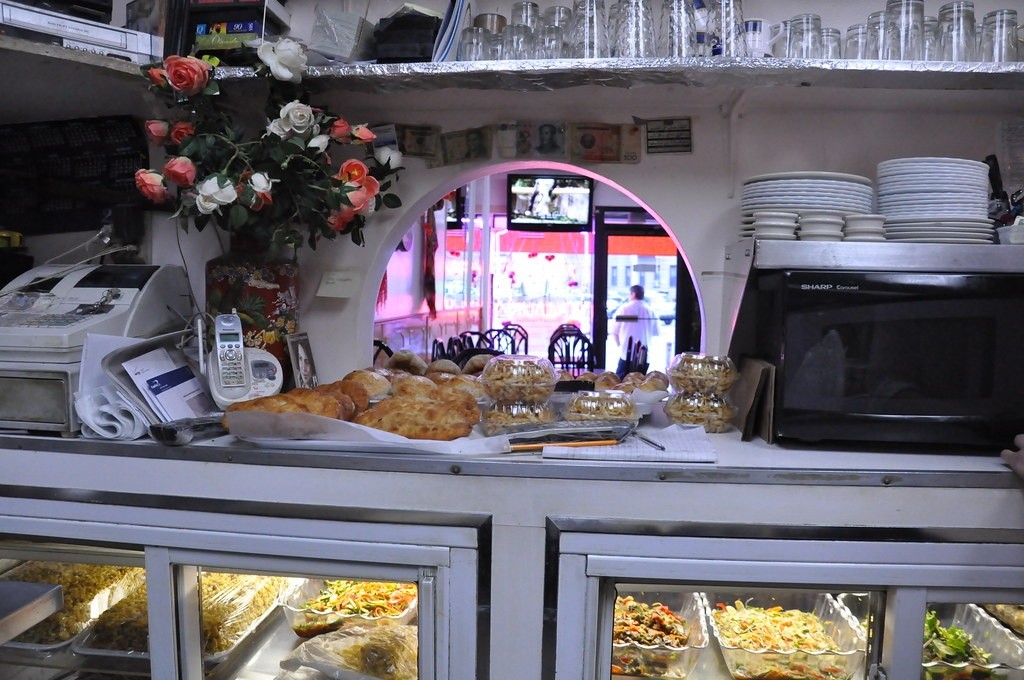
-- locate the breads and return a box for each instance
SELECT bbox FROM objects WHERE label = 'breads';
[220,352,670,440]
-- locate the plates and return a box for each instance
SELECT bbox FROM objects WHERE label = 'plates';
[738,156,995,244]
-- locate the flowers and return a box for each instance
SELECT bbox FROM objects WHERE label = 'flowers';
[133,36,404,264]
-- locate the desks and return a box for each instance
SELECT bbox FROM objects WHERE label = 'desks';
[553,368,607,379]
[552,355,597,364]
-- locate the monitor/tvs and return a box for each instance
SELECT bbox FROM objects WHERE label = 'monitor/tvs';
[506,173,594,233]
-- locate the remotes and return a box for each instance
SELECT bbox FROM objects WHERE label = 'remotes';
[149,415,228,443]
[491,420,632,445]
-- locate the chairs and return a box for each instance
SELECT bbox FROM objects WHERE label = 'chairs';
[373,323,650,380]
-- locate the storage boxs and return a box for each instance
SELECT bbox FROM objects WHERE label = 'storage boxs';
[194,19,278,50]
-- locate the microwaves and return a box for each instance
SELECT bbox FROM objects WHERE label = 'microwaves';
[755,243,1024,455]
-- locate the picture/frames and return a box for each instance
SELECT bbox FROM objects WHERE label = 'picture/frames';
[283,332,319,389]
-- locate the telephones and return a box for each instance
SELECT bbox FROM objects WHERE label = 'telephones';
[197,308,283,411]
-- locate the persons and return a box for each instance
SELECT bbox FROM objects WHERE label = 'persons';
[298,341,316,387]
[612,285,660,380]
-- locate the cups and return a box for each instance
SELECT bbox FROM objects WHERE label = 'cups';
[458,0,1024,61]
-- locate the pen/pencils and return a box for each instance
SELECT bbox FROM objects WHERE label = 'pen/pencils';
[631,430,667,450]
[509,439,623,451]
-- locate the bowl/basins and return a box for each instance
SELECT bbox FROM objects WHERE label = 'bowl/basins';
[996,225,1024,244]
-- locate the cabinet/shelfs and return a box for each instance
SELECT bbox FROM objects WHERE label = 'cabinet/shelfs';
[543,516,1024,680]
[1,486,492,680]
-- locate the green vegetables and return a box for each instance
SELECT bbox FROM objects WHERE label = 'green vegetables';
[857,610,1007,680]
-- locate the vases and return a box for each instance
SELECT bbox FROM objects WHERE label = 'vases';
[205,257,299,389]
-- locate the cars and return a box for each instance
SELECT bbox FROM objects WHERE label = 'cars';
[606,289,675,325]
